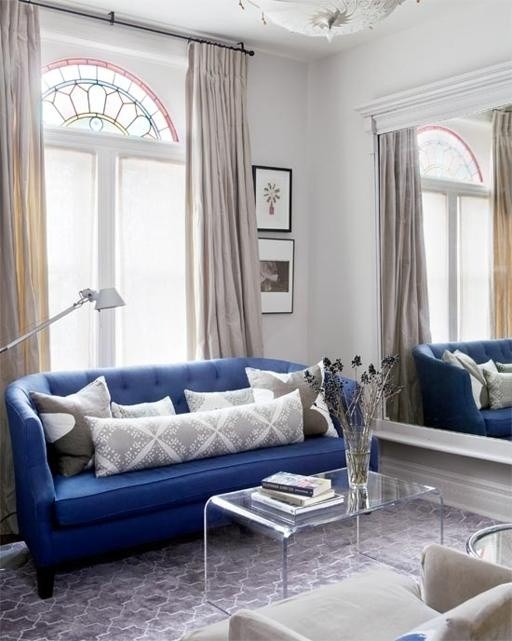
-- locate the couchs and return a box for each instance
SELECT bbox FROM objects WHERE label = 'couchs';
[5,357,377,599]
[412,339,512,438]
[178,545,512,640]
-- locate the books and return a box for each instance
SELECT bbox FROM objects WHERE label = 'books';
[250,470,345,516]
[250,501,345,526]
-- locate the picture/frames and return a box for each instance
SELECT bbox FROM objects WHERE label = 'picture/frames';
[252,165,292,233]
[256,237,295,314]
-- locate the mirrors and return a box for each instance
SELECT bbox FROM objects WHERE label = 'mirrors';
[352,60,512,463]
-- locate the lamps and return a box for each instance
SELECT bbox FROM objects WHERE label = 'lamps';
[238,0,420,40]
[1,287,127,354]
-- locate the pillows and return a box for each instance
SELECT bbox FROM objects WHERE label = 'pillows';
[28,374,113,478]
[441,349,488,411]
[183,388,254,412]
[244,361,339,439]
[477,359,499,381]
[110,396,178,419]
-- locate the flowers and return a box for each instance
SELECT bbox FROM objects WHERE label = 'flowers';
[303,355,408,477]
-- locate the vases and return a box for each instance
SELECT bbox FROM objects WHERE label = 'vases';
[343,427,373,489]
